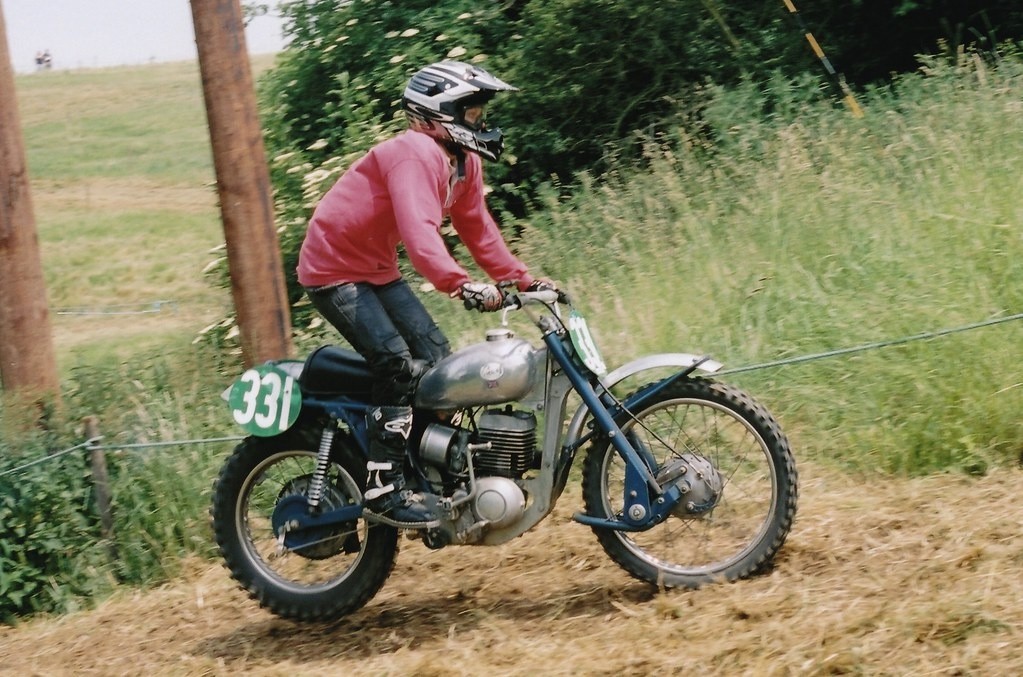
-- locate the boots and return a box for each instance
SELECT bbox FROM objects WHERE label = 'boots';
[361,406,441,530]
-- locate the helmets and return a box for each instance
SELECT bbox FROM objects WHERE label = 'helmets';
[401,60,520,163]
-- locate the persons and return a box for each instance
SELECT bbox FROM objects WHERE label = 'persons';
[295,60,558,529]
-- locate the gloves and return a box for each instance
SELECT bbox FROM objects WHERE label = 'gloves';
[526,279,571,306]
[458,281,503,312]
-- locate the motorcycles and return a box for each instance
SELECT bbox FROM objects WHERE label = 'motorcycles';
[208,278,801,623]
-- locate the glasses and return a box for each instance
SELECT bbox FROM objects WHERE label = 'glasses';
[464,103,489,122]
